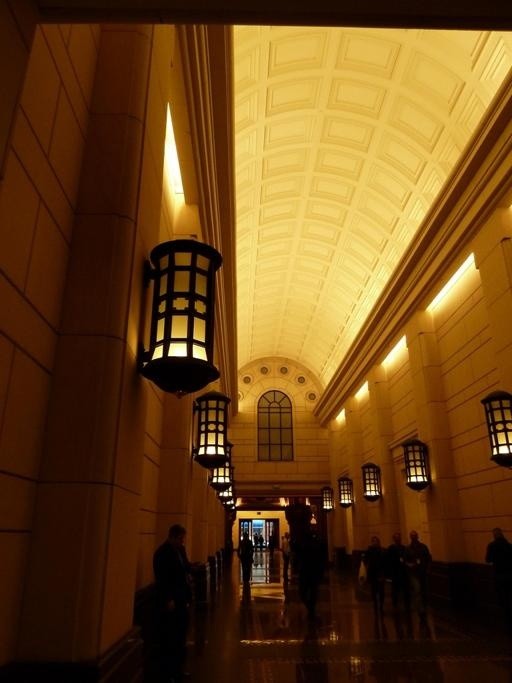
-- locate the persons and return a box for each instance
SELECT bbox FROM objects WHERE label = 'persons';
[386,533,414,614]
[406,531,433,612]
[153,524,202,675]
[359,537,388,620]
[236,504,324,619]
[485,528,512,566]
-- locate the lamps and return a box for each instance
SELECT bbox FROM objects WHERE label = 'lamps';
[321,486,333,512]
[481,389,512,466]
[193,390,231,468]
[338,476,353,507]
[209,439,236,513]
[137,240,222,398]
[402,438,431,490]
[361,462,380,497]
